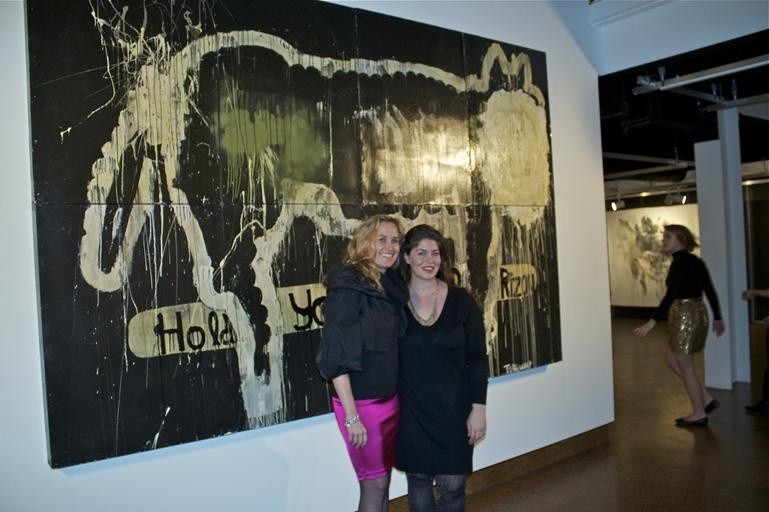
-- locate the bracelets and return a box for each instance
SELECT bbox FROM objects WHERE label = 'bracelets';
[344,414,359,427]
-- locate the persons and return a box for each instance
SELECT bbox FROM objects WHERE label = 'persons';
[391,224,489,512]
[317,214,410,512]
[632,224,725,427]
[741,289,769,414]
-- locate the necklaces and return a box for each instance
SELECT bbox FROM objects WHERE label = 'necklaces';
[409,278,439,326]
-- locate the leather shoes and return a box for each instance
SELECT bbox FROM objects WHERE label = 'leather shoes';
[674,414,709,426]
[703,398,721,414]
